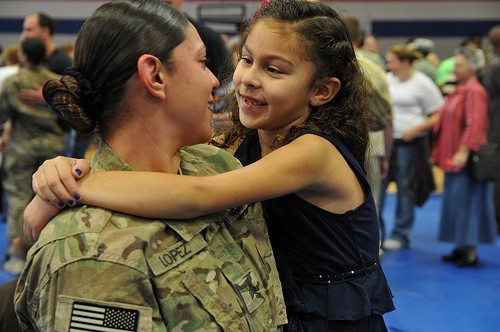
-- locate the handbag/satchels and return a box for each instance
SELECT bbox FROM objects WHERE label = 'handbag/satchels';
[467,150,491,181]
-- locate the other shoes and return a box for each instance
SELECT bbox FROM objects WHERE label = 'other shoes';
[382,238,404,251]
[3,259,25,275]
[441,246,478,268]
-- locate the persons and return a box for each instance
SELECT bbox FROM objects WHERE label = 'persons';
[0,5,499,276]
[21,1,396,332]
[12,1,288,332]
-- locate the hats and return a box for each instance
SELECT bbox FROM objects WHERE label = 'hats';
[414,38,434,52]
